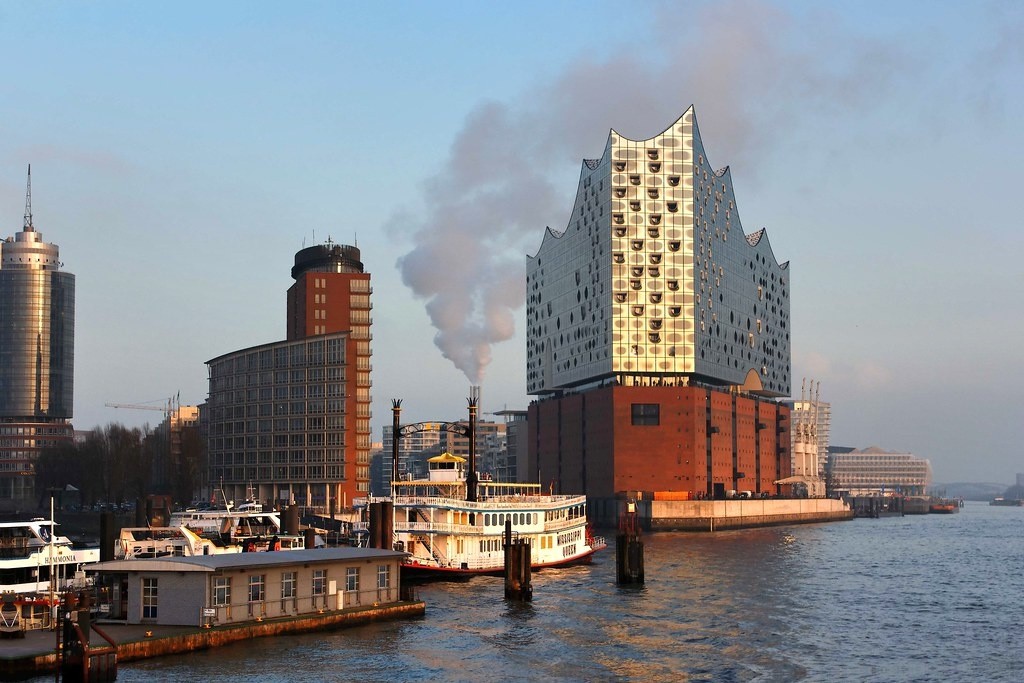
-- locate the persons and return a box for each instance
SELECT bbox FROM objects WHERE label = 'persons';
[688,490,692,500]
[761,491,764,497]
[708,493,711,500]
[752,492,754,497]
[698,494,703,499]
[744,493,747,499]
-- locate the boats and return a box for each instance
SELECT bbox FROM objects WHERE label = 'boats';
[903,497,958,516]
[0,496,86,637]
[989,494,1023,507]
[366,387,611,575]
[114,475,306,559]
